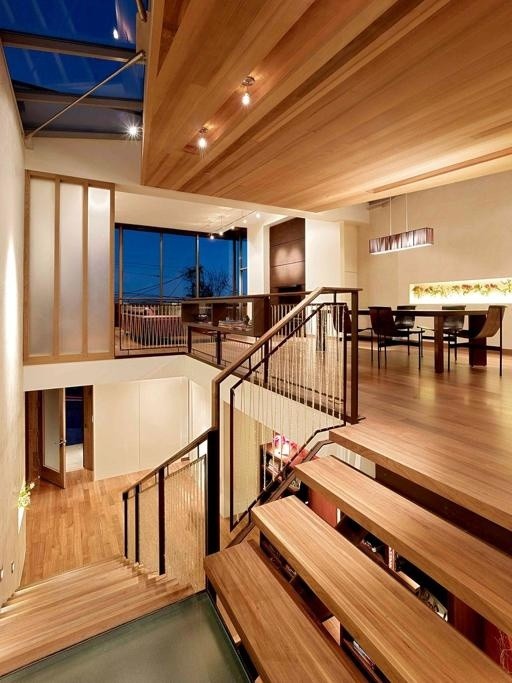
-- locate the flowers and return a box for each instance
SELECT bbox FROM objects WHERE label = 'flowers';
[17,476,40,510]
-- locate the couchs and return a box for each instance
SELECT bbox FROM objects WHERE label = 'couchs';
[122,314,183,337]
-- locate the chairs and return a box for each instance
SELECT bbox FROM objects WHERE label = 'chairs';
[331,305,507,377]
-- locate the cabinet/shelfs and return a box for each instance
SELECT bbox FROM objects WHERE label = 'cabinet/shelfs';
[340,534,480,683]
[260,439,320,582]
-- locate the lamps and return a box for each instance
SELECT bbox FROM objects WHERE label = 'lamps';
[369,194,433,255]
[208,210,260,240]
[198,127,208,148]
[242,77,255,105]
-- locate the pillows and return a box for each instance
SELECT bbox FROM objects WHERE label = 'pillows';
[144,308,154,315]
